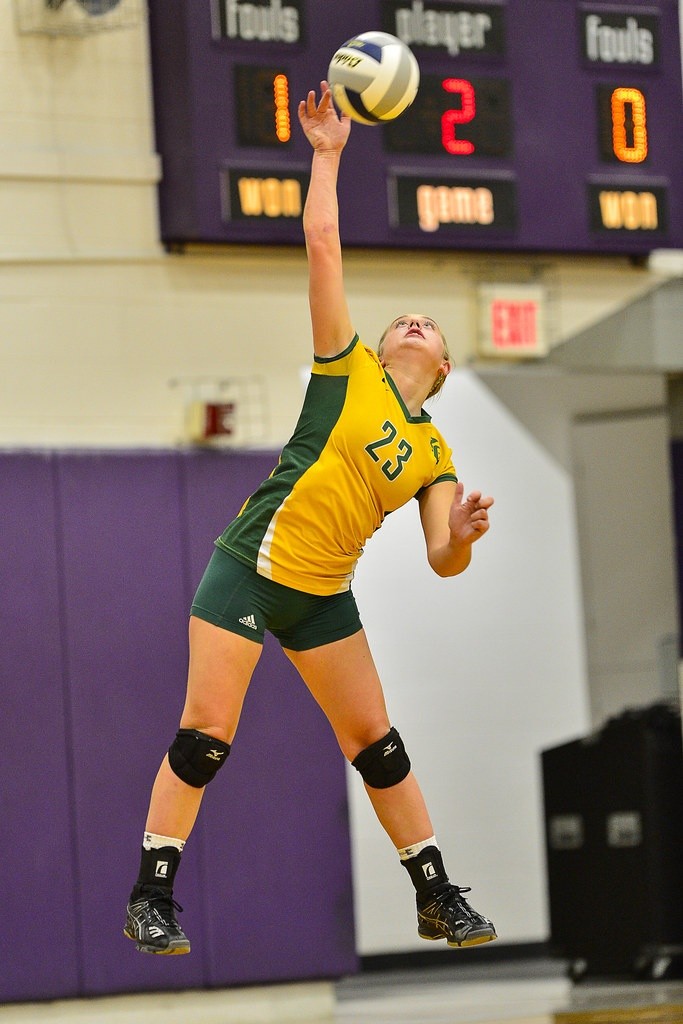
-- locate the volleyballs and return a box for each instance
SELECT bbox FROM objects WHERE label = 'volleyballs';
[326,27,424,128]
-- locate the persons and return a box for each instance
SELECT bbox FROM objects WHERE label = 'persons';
[124,76,505,956]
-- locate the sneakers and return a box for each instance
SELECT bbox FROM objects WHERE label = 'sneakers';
[416,881,497,947]
[124,887,191,956]
